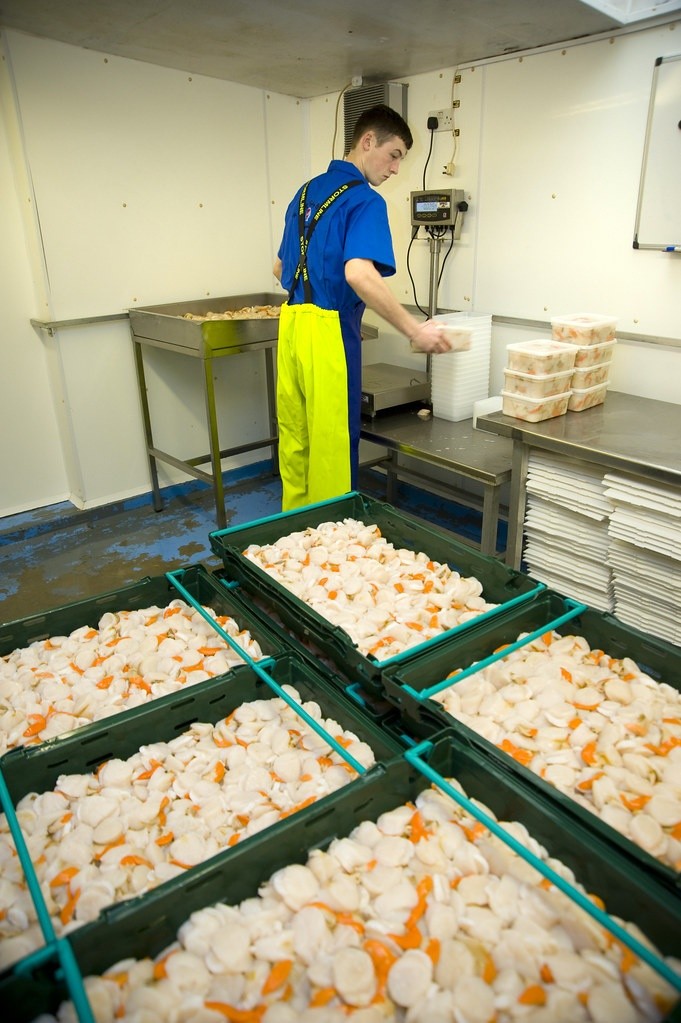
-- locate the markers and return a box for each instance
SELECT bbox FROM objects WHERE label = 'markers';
[666,245,681,252]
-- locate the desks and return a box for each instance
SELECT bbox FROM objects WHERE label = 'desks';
[360,401,513,564]
[475,388,681,650]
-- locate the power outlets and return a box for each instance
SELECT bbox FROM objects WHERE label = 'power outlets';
[425,108,454,132]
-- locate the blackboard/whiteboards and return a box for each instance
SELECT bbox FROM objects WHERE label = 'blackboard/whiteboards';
[633,53,681,253]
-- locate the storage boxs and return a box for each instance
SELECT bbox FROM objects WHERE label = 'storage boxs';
[0,484,681,1023]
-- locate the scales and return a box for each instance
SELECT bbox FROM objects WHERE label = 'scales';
[362,187,467,417]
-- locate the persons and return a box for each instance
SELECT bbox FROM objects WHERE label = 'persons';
[270,104,454,513]
